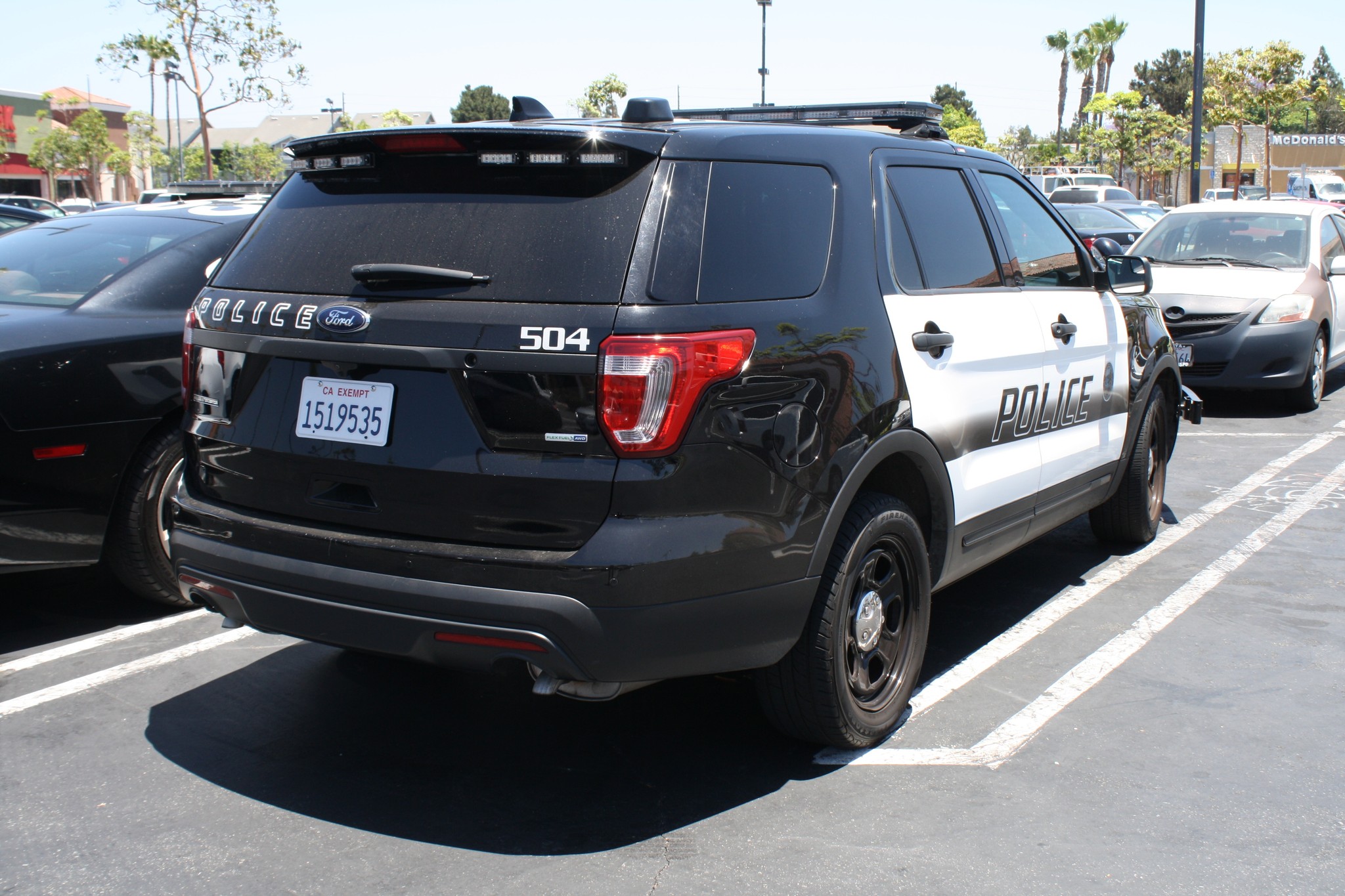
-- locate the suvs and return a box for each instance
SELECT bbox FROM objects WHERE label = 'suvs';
[163,96,1184,752]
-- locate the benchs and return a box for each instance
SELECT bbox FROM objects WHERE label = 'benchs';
[1232,235,1287,258]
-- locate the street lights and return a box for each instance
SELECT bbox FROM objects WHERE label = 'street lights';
[755,0,773,107]
[1078,85,1098,124]
[164,60,184,182]
[321,98,342,134]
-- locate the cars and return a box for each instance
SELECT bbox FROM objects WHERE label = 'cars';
[0,203,266,612]
[0,180,286,238]
[1091,199,1345,411]
[1033,156,1345,259]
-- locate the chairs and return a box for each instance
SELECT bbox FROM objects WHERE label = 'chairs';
[1284,230,1307,258]
[1195,224,1237,257]
